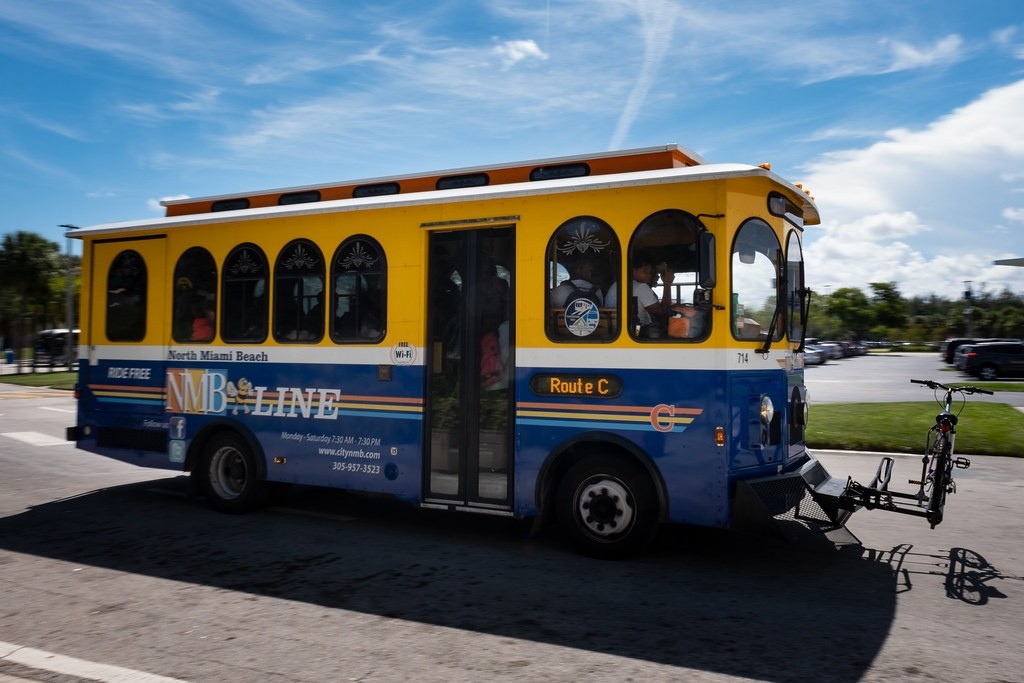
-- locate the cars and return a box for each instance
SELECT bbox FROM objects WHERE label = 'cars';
[939,337,1024,381]
[803,337,902,363]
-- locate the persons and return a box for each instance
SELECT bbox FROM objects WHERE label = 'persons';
[604,251,674,326]
[191,296,216,343]
[431,259,509,475]
[549,253,603,308]
[281,288,384,341]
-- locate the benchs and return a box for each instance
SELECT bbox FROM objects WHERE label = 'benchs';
[548,307,618,341]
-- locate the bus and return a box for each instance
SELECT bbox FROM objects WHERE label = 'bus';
[33,329,79,367]
[65,143,897,530]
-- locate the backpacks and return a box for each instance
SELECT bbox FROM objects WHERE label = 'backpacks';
[560,280,602,309]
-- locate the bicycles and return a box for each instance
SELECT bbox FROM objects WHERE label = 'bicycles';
[910,379,996,525]
